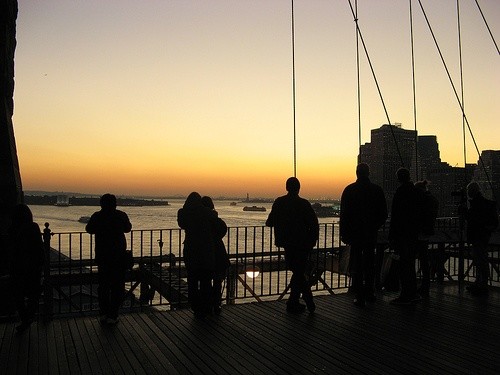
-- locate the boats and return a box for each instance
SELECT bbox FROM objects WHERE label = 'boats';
[243,205,268,212]
[78,216,91,223]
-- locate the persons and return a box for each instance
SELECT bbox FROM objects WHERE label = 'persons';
[176,191,219,319]
[338,162,389,304]
[391,168,440,305]
[197,196,229,314]
[464,181,500,297]
[2,204,46,329]
[266,176,321,314]
[84,193,133,326]
